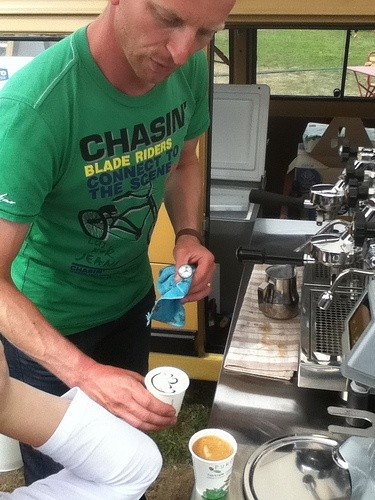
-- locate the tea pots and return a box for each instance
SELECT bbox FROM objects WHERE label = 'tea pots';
[258,263,299,320]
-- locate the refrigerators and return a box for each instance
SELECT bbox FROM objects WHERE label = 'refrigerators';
[208,84,270,322]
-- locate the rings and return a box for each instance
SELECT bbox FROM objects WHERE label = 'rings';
[207,282,211,287]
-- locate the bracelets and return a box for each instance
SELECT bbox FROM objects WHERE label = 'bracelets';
[175,228,206,245]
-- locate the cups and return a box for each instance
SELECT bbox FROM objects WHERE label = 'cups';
[188,427,238,500]
[144,367,190,418]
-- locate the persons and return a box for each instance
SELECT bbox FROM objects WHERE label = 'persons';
[0,0,238,486]
[0,339,163,500]
